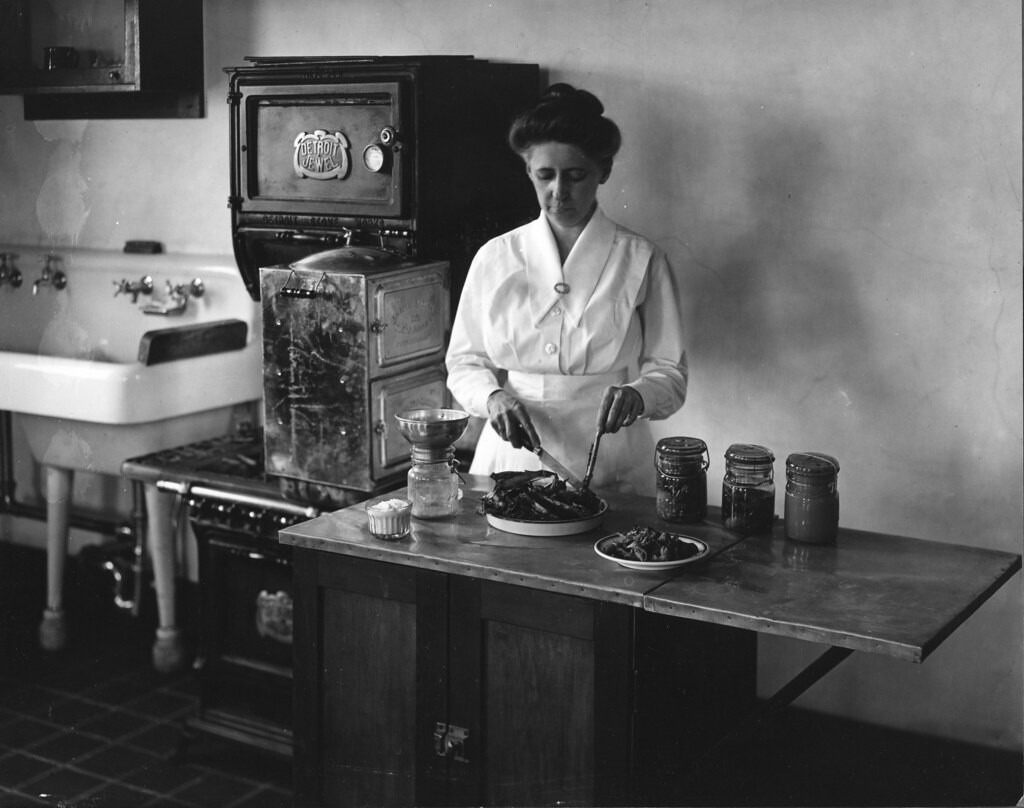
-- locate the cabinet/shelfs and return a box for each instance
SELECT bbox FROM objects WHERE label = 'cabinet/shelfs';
[122,53,541,763]
[0,0,203,119]
[279,470,1022,808]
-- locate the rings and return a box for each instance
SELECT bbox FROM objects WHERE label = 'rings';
[497,428,505,433]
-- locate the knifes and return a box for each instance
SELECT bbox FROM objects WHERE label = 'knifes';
[517,429,601,508]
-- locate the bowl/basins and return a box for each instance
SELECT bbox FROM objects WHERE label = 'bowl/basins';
[365,497,413,540]
[395,409,471,452]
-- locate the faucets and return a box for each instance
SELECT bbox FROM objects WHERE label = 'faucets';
[30,255,68,296]
[160,275,205,314]
[111,274,153,304]
[0,251,23,289]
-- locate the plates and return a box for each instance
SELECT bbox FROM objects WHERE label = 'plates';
[594,531,710,570]
[487,498,608,536]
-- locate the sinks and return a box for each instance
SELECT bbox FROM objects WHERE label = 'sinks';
[0,243,263,476]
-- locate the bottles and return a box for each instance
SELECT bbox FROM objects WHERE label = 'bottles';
[654,436,710,521]
[407,459,465,520]
[783,452,840,543]
[721,443,775,533]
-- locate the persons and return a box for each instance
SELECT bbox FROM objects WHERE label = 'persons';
[444,84,690,496]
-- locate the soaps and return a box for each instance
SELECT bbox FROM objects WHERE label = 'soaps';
[126,240,157,247]
